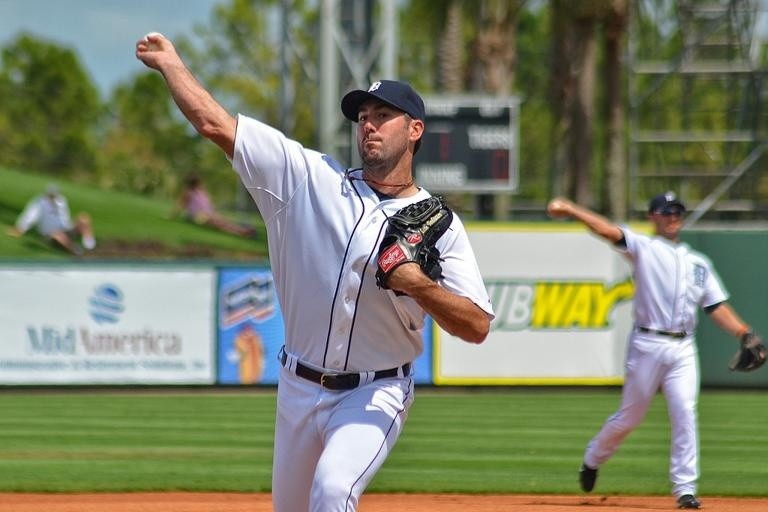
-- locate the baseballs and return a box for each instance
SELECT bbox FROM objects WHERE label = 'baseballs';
[142,32,164,51]
[548,200,562,210]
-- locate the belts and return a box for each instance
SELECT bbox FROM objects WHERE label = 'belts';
[636,324,688,340]
[277,345,413,392]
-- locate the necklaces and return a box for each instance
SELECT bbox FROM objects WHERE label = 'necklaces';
[344,164,417,194]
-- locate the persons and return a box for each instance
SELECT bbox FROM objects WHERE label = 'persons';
[160,173,257,240]
[544,191,768,508]
[3,182,97,255]
[132,31,496,510]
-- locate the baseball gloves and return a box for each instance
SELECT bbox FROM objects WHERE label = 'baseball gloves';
[375,196,454,296]
[727,332,767,371]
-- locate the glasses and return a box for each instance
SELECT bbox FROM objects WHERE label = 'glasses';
[654,210,681,218]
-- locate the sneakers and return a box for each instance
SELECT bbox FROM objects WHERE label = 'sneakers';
[677,494,701,508]
[577,461,599,493]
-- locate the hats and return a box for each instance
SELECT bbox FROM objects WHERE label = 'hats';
[340,78,427,128]
[649,189,686,214]
